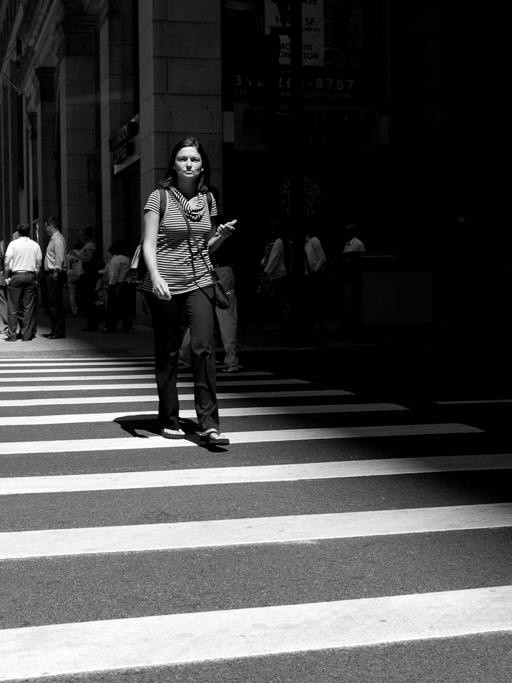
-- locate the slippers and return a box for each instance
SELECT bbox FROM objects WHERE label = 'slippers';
[197,428,230,447]
[158,417,188,440]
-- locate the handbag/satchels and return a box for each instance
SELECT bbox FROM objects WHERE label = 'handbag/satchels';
[82,247,106,273]
[120,180,171,294]
[210,274,237,311]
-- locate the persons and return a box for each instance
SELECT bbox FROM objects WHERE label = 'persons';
[143,137,237,447]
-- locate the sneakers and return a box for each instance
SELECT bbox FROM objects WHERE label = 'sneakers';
[176,348,192,368]
[221,355,241,372]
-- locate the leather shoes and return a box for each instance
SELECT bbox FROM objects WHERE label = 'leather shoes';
[0,323,136,342]
